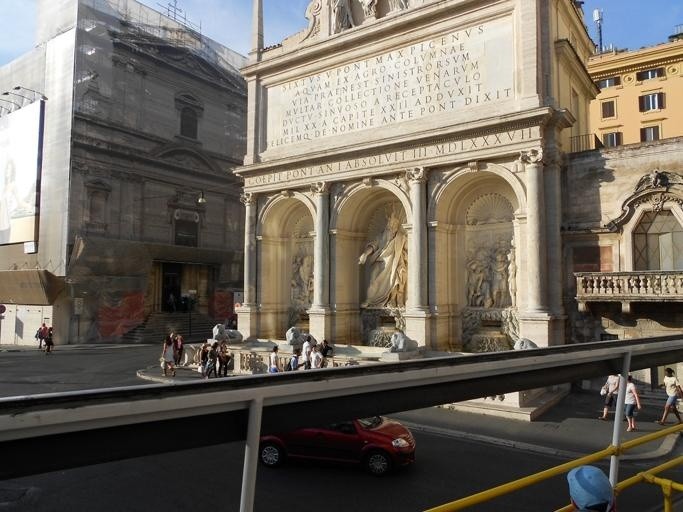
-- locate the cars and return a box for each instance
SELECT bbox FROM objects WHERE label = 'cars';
[255,413,420,476]
[224,314,237,330]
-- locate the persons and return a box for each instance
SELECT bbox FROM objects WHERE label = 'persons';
[466,249,516,308]
[270,335,333,373]
[624,376,641,432]
[358,217,409,308]
[38,323,54,352]
[160,332,231,380]
[598,375,620,421]
[655,368,683,425]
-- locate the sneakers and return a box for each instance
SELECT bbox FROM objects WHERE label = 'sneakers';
[598,414,683,432]
